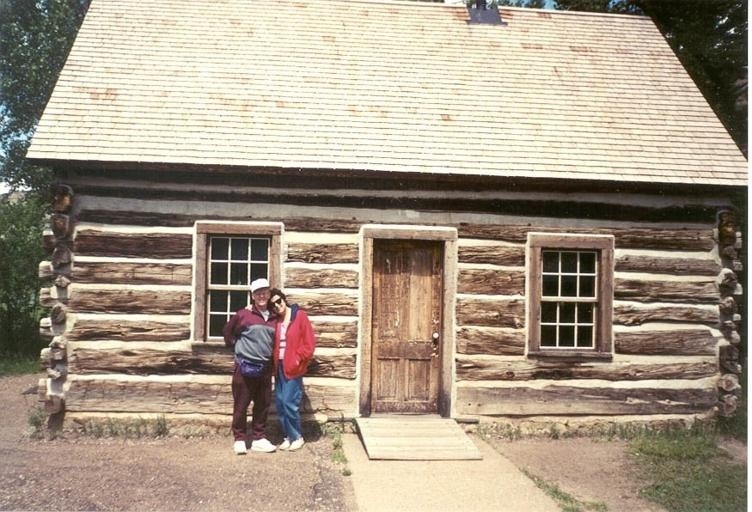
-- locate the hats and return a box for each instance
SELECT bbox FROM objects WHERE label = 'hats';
[250,278,270,293]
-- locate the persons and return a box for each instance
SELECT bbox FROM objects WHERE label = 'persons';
[244,288,315,451]
[223,278,278,454]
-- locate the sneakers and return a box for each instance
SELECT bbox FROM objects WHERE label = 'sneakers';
[278,436,305,450]
[234,439,248,454]
[250,437,277,453]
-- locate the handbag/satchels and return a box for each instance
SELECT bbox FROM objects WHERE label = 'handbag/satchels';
[235,355,272,378]
